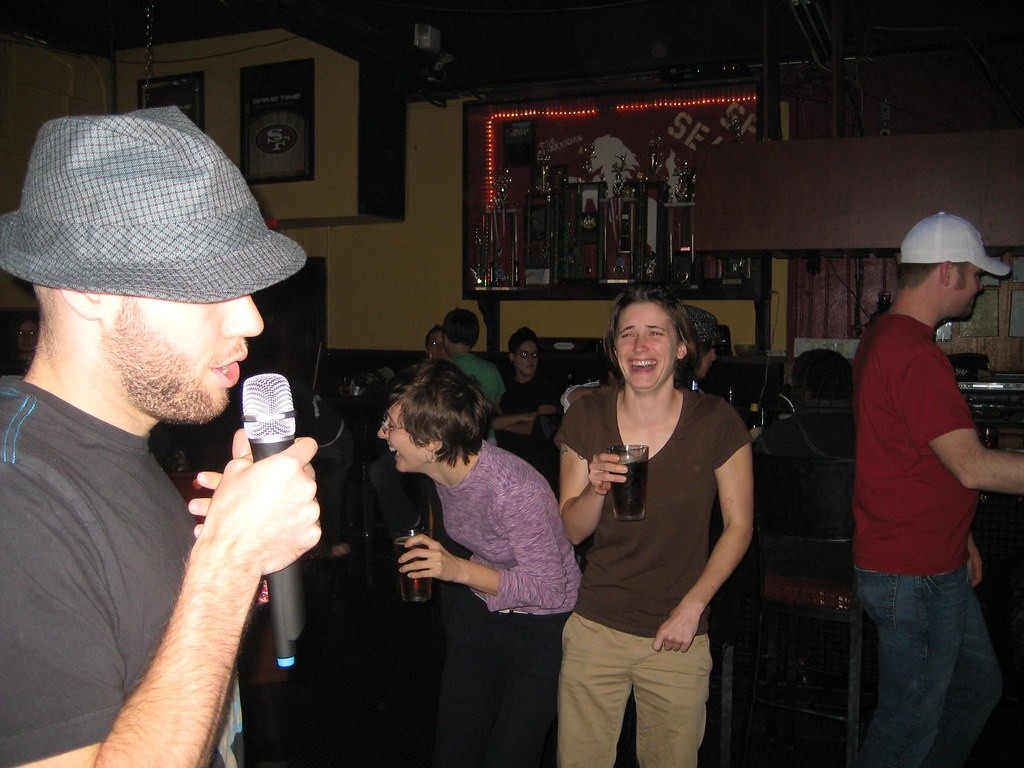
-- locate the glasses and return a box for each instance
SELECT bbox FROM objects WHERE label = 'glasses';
[516,351,538,360]
[381,421,404,435]
[428,340,439,351]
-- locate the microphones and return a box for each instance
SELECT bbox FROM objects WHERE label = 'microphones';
[243,374,307,667]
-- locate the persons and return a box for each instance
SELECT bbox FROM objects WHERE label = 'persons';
[852,211,1024,768]
[755,348,853,539]
[378,362,583,768]
[419,310,506,410]
[6,310,40,376]
[335,364,404,406]
[424,325,447,360]
[490,327,563,465]
[2,105,322,767]
[557,288,755,768]
[687,304,722,393]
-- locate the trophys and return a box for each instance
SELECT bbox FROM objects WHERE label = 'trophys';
[470,119,751,291]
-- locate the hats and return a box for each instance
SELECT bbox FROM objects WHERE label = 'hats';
[683,304,719,342]
[0,106,308,303]
[900,212,1011,276]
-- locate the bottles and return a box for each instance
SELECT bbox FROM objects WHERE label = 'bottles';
[341,377,351,395]
[562,373,576,394]
[721,384,762,440]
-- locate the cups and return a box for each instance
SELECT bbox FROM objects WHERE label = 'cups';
[607,446,648,521]
[388,528,433,602]
[429,341,445,358]
[980,425,998,450]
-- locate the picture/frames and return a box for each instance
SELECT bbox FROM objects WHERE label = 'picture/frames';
[238,58,317,186]
[137,71,206,129]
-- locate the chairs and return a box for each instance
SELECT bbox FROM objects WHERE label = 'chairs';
[719,453,879,768]
